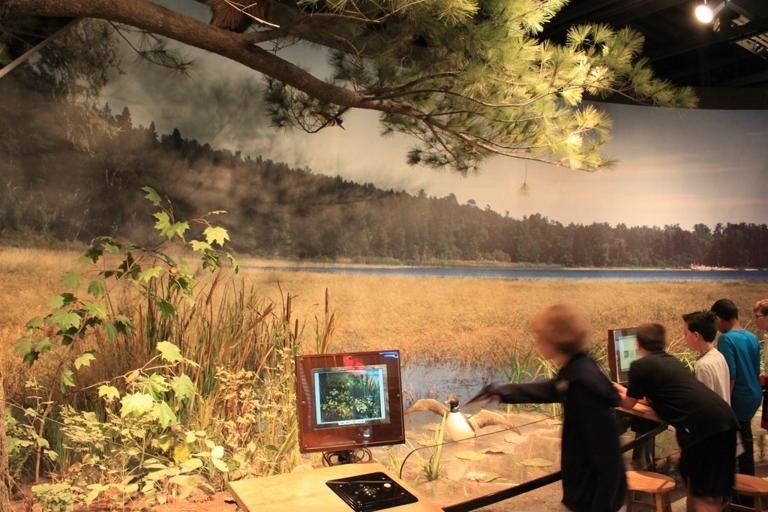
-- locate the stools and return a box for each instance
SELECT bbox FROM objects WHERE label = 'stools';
[623,471,767,512]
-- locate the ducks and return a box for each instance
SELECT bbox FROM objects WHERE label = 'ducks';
[403,391,525,447]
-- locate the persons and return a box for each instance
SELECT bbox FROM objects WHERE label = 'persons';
[490,303,630,512]
[610,322,739,512]
[753,299,768,429]
[679,308,733,407]
[712,299,764,477]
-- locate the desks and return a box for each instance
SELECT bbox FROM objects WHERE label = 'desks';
[231,460,444,511]
